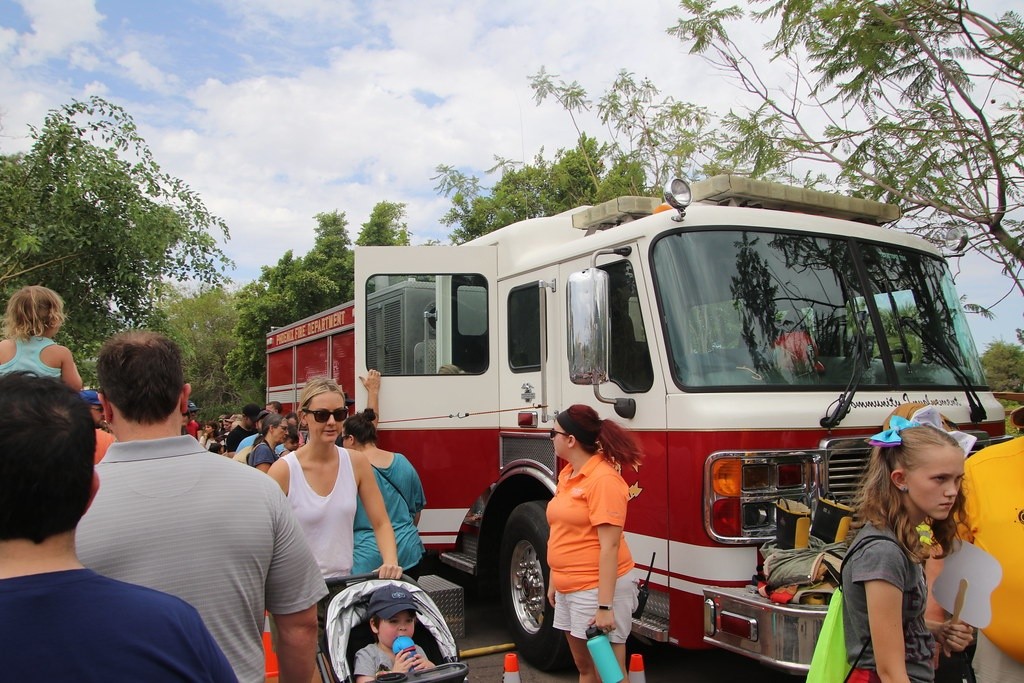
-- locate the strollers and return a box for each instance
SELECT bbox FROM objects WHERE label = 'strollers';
[315,570,470,683]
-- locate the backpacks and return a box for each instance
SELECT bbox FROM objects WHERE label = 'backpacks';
[804,535,910,683]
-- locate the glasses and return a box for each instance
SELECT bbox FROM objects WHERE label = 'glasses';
[190,410,196,414]
[303,408,348,423]
[91,407,103,413]
[550,428,568,439]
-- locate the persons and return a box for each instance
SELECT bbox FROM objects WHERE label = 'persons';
[359,369,381,428]
[266,377,403,683]
[181,400,305,474]
[922,436,1024,683]
[0,370,238,683]
[354,584,436,683]
[0,285,82,391]
[545,405,640,683]
[840,414,973,683]
[75,329,329,683]
[78,390,115,512]
[343,409,426,582]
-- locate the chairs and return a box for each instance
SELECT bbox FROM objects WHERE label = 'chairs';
[610,302,653,388]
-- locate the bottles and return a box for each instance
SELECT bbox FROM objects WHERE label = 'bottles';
[393,635,419,672]
[585,625,625,683]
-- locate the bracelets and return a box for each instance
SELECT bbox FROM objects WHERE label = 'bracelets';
[597,604,612,610]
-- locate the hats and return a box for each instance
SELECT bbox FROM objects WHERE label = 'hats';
[187,400,199,412]
[224,418,235,423]
[368,586,423,619]
[243,404,260,418]
[79,390,100,404]
[218,415,228,421]
[257,410,269,420]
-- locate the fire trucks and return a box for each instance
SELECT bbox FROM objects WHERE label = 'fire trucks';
[263,174,1017,677]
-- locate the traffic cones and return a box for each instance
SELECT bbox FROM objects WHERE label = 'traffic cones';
[627,653,647,683]
[262,608,278,679]
[502,653,523,683]
